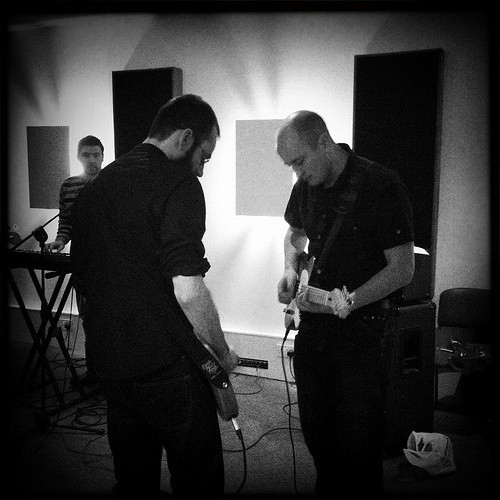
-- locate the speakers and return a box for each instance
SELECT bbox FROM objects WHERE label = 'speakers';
[112,66,183,158]
[353,48,447,462]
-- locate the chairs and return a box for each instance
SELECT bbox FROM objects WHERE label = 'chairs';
[436,288,490,415]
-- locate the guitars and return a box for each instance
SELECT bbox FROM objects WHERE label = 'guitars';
[284,252,354,330]
[200,351,238,420]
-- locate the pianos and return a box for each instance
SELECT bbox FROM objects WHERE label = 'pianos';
[4,249,102,415]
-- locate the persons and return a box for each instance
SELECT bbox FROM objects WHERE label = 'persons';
[70,94,240,500]
[45,135,103,387]
[275,111,416,500]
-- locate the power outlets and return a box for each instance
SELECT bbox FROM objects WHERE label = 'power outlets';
[278,345,295,358]
[59,319,73,331]
[238,358,268,370]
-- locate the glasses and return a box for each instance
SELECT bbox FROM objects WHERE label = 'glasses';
[199,145,210,164]
